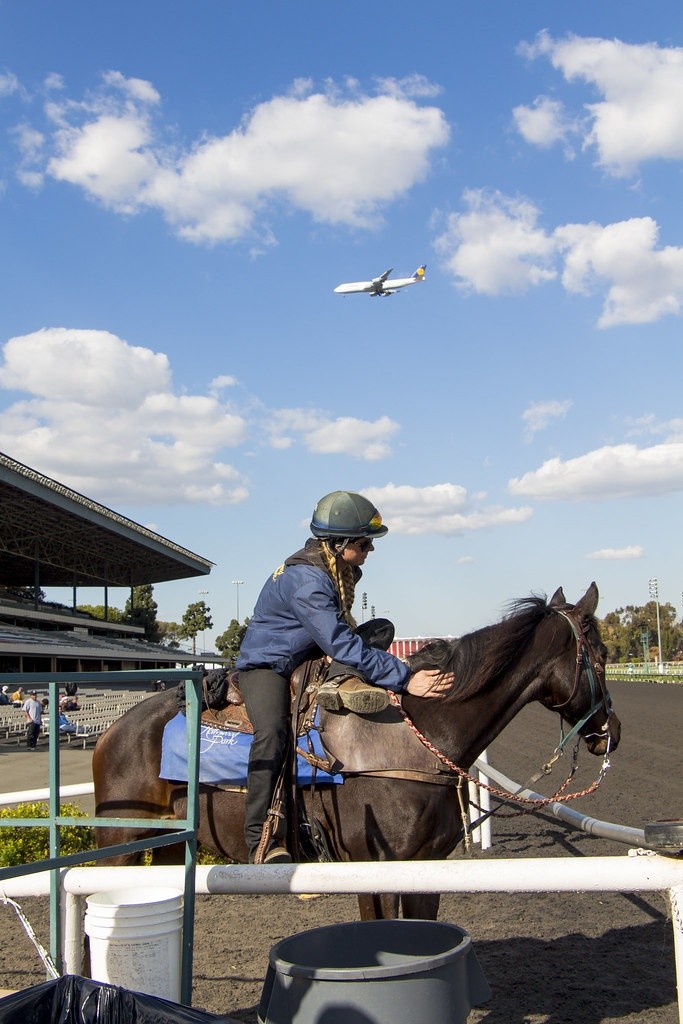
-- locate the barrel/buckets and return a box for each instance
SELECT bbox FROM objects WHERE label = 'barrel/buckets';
[257,918,493,1024]
[83,890,183,1004]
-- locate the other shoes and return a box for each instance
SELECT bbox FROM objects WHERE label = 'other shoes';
[27,748,39,751]
[264,847,292,864]
[316,675,390,715]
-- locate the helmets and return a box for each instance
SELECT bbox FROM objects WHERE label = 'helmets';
[310,490,389,540]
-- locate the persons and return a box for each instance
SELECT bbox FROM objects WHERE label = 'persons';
[0,685,25,709]
[235,490,455,864]
[59,695,82,712]
[35,698,49,714]
[58,706,92,734]
[22,690,41,752]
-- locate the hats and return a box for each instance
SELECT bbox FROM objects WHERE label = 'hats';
[30,690,38,695]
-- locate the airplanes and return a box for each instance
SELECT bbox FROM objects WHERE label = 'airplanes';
[333,264,427,297]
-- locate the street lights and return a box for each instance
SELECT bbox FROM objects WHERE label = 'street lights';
[232,581,244,621]
[198,591,209,652]
[648,578,664,674]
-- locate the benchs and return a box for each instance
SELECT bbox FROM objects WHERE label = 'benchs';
[0,687,163,750]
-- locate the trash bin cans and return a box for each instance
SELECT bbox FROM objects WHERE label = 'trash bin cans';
[0,974,243,1024]
